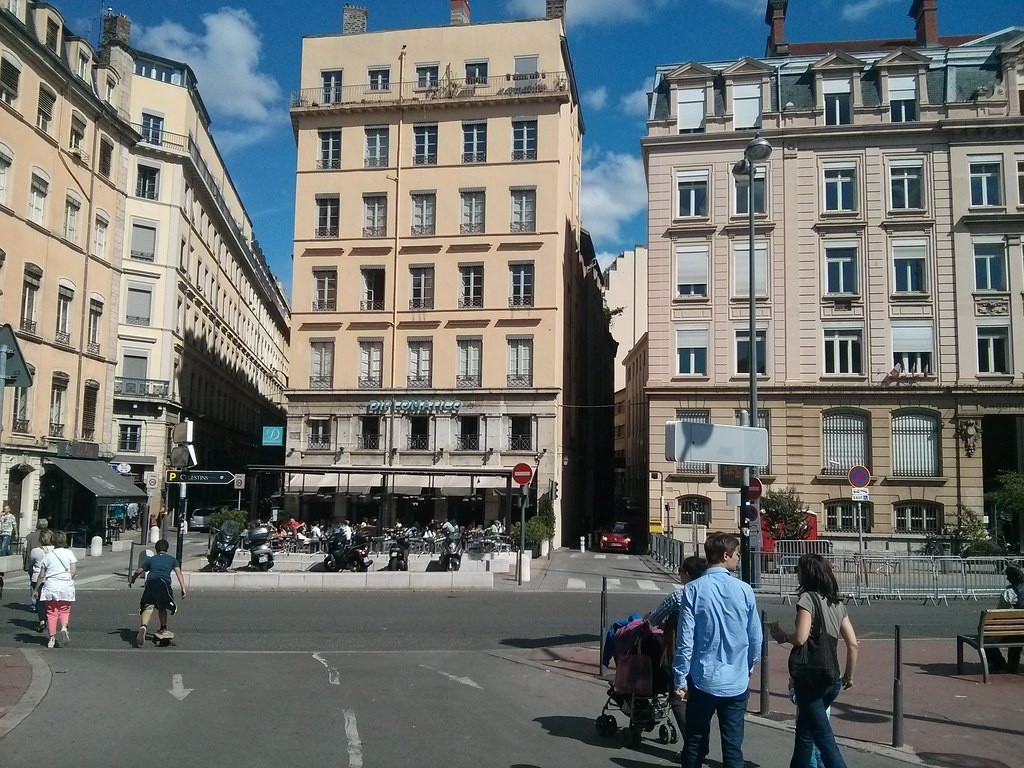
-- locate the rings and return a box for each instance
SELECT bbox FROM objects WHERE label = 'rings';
[772,632,773,636]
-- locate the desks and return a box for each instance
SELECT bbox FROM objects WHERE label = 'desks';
[67,530,79,547]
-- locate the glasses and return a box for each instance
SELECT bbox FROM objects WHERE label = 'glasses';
[794,564,801,573]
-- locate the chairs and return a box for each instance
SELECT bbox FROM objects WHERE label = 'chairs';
[270,526,512,555]
[80,524,90,544]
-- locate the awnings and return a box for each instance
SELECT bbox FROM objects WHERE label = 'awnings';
[44,459,148,505]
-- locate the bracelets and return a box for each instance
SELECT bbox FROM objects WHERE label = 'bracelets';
[783,634,787,644]
[844,673,853,681]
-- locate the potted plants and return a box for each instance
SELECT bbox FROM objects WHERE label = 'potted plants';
[512,498,556,557]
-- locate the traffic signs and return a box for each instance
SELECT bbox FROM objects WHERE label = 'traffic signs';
[166,469,237,485]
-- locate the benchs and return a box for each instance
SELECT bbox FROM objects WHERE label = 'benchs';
[957,609,1024,684]
[778,555,802,576]
[842,550,902,576]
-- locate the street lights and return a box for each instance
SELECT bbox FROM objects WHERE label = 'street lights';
[731,134,773,596]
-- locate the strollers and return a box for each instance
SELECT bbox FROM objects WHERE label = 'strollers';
[594,611,680,746]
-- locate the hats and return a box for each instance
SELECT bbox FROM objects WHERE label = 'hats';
[495,520,501,524]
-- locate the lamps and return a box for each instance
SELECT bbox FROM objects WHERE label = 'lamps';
[339,446,344,453]
[543,448,547,453]
[563,455,569,467]
[891,362,901,378]
[490,448,494,451]
[290,448,294,452]
[393,447,397,453]
[785,102,795,110]
[440,448,444,452]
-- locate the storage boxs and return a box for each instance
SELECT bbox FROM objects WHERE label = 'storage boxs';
[250,525,269,542]
[354,527,373,545]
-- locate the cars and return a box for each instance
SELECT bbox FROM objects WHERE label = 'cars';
[599,521,635,553]
[188,507,215,533]
[615,495,642,514]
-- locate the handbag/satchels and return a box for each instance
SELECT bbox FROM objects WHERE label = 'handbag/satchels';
[787,591,840,685]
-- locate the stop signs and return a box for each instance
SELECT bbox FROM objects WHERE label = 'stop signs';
[513,462,533,485]
[748,476,762,500]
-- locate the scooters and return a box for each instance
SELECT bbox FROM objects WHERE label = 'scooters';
[387,530,410,571]
[246,526,275,572]
[207,526,243,572]
[322,535,372,572]
[439,532,465,572]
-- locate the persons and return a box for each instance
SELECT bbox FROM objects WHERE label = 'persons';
[672,532,763,768]
[644,556,708,757]
[267,518,352,545]
[984,564,1024,673]
[360,516,506,537]
[150,514,157,526]
[769,554,858,768]
[23,519,78,648]
[0,505,18,556]
[127,539,187,645]
[76,519,88,542]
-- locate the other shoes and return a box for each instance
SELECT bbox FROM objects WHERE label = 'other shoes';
[61,629,70,645]
[989,663,1007,673]
[136,626,147,645]
[157,628,167,635]
[36,625,45,633]
[48,636,56,650]
